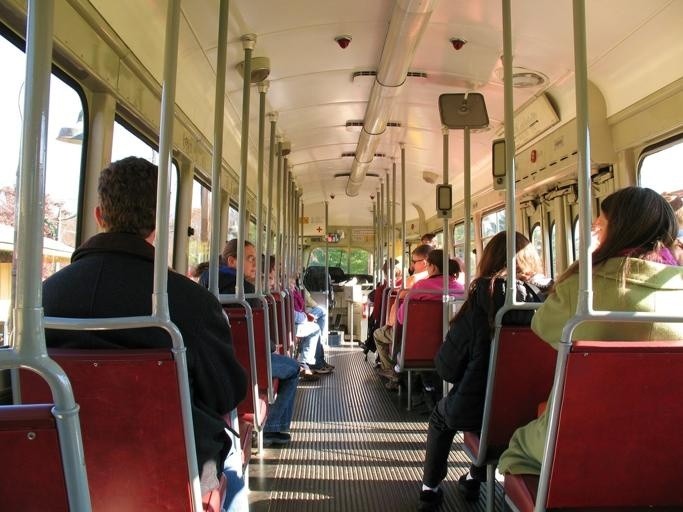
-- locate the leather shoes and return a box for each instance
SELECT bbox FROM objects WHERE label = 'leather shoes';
[263,432,292,447]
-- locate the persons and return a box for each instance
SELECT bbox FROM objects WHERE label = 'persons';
[361,235,465,409]
[421,230,553,505]
[193,239,335,444]
[498,187,683,475]
[40,157,247,512]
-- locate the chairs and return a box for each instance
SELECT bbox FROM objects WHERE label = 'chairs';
[365,285,683,510]
[0,280,305,510]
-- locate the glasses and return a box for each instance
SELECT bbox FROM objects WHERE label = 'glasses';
[245,254,256,264]
[410,258,427,265]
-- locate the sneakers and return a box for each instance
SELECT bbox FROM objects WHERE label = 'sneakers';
[416,487,443,512]
[458,473,480,505]
[298,362,334,382]
